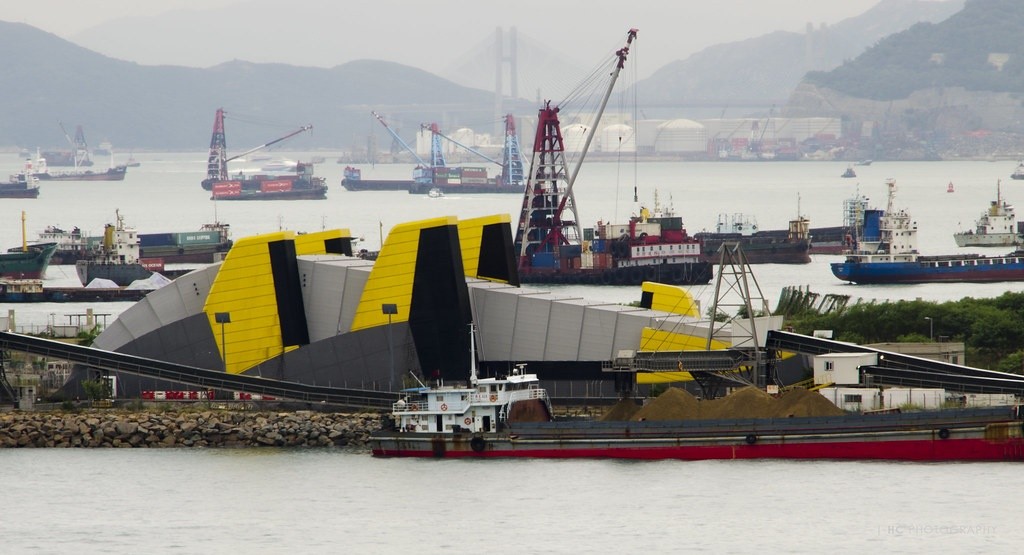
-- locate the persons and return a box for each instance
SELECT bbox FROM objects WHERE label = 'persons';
[678,360,682,371]
[1012,401,1018,419]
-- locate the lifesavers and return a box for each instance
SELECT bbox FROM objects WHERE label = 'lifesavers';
[745,433,757,444]
[411,403,418,411]
[470,436,485,451]
[464,418,471,425]
[490,395,496,402]
[440,404,447,411]
[938,428,950,440]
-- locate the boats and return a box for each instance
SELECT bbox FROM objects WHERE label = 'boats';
[0,210,233,303]
[201,105,328,200]
[693,191,813,265]
[809,181,886,255]
[953,178,1024,247]
[408,114,526,194]
[830,178,1024,284]
[514,27,713,286]
[367,322,1024,464]
[0,119,141,199]
[341,110,446,191]
